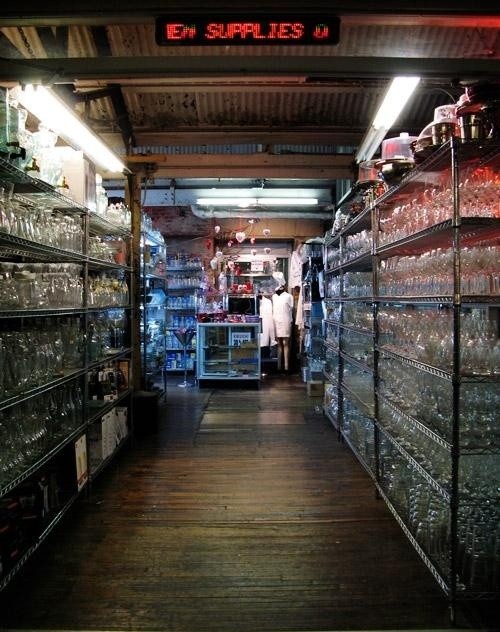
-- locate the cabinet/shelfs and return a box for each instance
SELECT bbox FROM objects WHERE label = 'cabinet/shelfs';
[165,251,199,375]
[198,321,263,382]
[215,253,261,314]
[0,165,165,590]
[301,135,500,621]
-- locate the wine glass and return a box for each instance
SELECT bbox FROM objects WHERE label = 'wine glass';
[322,163,500,586]
[0,171,129,479]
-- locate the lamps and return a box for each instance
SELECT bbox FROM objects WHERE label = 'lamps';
[196,198,320,206]
[10,85,127,175]
[353,74,423,164]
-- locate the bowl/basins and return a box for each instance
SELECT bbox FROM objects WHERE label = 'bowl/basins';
[376,159,415,187]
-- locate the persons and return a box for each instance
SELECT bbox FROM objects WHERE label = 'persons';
[270,286,294,375]
[292,286,300,320]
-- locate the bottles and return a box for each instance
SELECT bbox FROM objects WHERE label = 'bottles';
[168,254,206,308]
[142,211,166,242]
